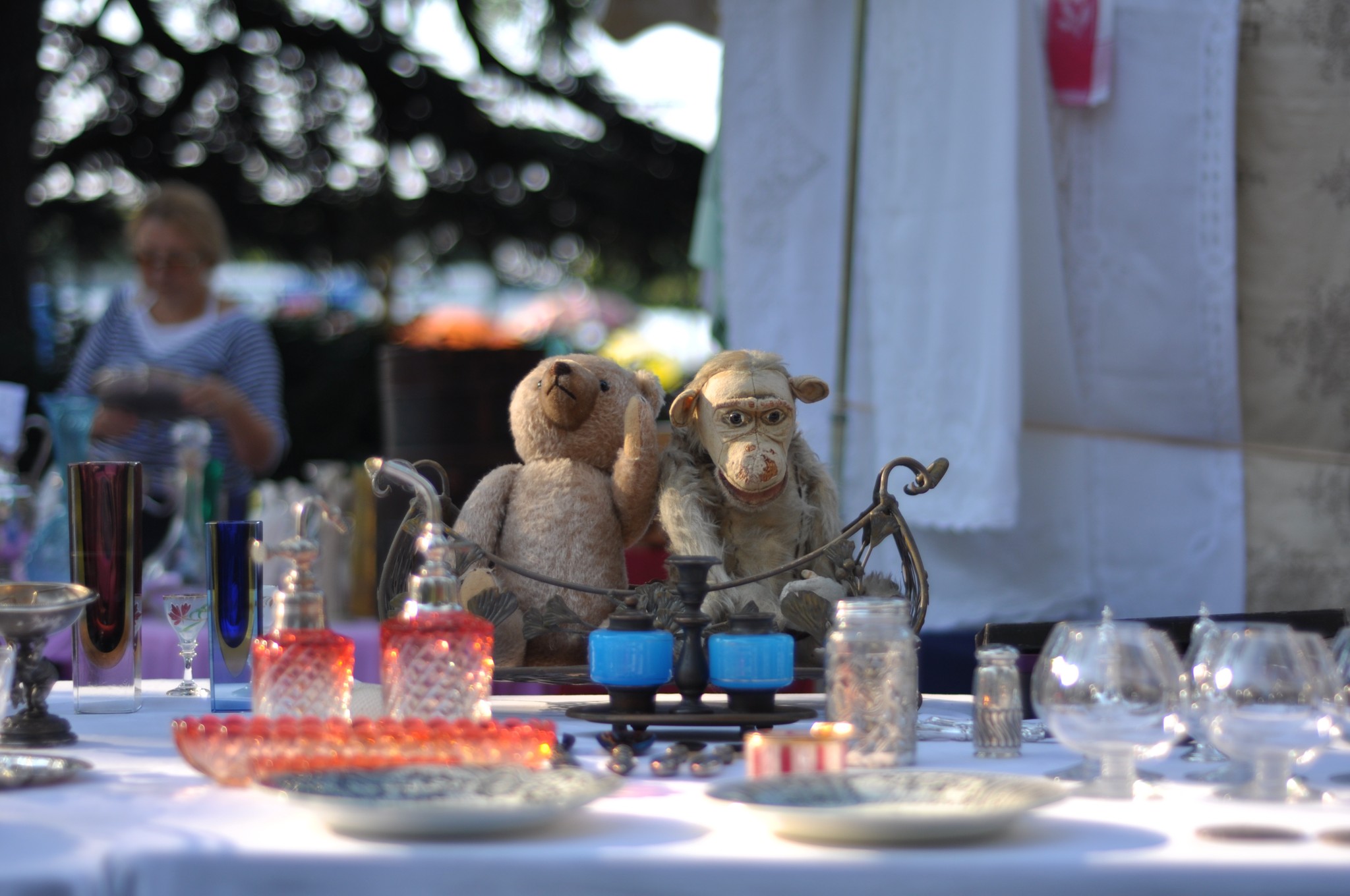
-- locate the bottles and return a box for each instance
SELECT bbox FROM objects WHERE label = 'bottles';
[824,593,919,772]
[362,456,495,722]
[252,495,354,722]
[970,640,1025,762]
[131,419,209,680]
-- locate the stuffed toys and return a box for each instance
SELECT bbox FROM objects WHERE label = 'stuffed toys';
[657,350,859,636]
[448,352,666,669]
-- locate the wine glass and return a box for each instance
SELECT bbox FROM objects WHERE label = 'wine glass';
[163,593,211,700]
[1028,619,1350,809]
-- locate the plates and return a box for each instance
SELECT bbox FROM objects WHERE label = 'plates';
[700,768,1071,844]
[174,714,556,791]
[255,765,617,839]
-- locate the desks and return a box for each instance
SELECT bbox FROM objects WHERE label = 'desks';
[0,676,1350,896]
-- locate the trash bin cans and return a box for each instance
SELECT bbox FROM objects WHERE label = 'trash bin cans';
[262,315,393,484]
[379,340,544,569]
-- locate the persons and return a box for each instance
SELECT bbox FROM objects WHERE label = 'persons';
[52,188,288,536]
